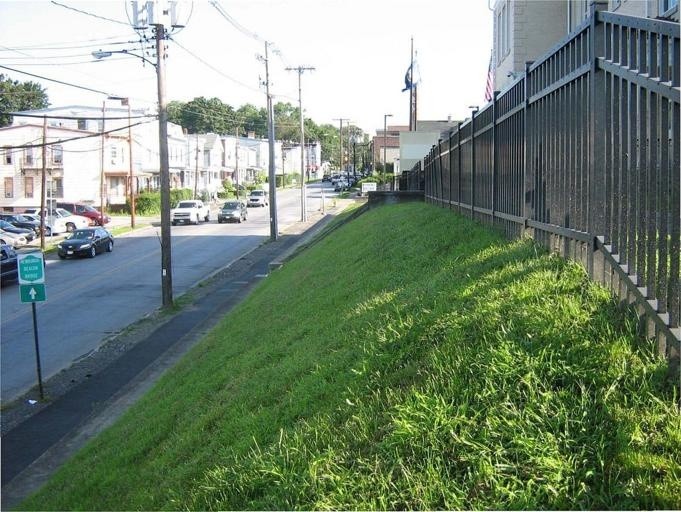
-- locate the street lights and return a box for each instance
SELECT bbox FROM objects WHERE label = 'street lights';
[89,46,177,311]
[383,113,394,192]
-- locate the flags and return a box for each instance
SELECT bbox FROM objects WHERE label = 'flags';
[402,59,421,91]
[485,51,493,101]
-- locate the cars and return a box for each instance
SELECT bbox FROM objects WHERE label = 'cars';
[0,198,117,289]
[321,173,356,192]
[246,189,269,208]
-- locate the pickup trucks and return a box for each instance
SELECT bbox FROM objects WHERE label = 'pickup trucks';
[171,199,211,226]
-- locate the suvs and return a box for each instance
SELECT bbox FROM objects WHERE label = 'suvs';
[217,200,248,225]
